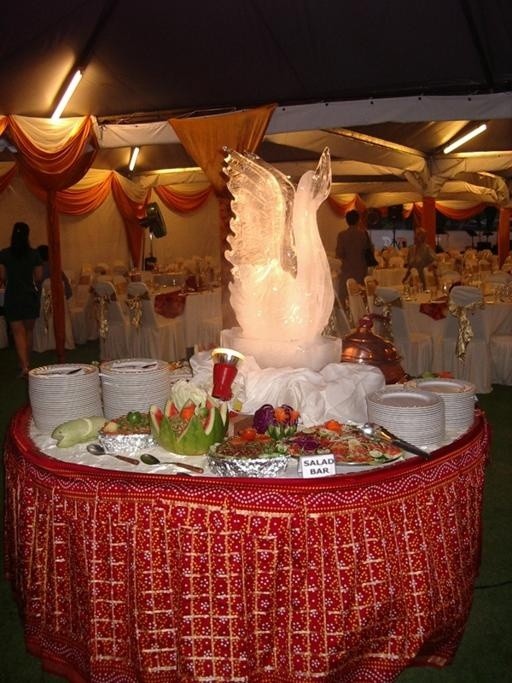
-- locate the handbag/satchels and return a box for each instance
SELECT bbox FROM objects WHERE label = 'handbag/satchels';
[363,231,378,266]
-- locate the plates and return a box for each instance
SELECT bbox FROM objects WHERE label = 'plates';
[366,373,473,445]
[305,422,404,467]
[152,271,186,291]
[29,358,171,431]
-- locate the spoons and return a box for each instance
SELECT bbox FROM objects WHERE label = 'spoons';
[140,453,204,473]
[112,365,157,371]
[35,368,84,376]
[88,444,140,463]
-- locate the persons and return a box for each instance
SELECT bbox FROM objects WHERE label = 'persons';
[1,220,41,380]
[335,210,377,295]
[32,243,71,301]
[402,226,436,286]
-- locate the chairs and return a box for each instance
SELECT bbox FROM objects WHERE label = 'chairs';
[0,241,511,394]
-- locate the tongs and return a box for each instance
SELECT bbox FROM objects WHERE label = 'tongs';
[360,421,430,461]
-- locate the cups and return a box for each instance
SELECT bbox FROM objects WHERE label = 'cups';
[400,278,509,302]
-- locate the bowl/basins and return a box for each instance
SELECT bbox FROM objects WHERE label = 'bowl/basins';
[209,442,288,478]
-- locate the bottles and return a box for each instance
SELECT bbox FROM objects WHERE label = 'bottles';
[209,348,246,401]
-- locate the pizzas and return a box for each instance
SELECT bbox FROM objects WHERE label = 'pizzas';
[291,418,401,466]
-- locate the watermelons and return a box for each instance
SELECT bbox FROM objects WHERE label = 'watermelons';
[143,398,229,455]
